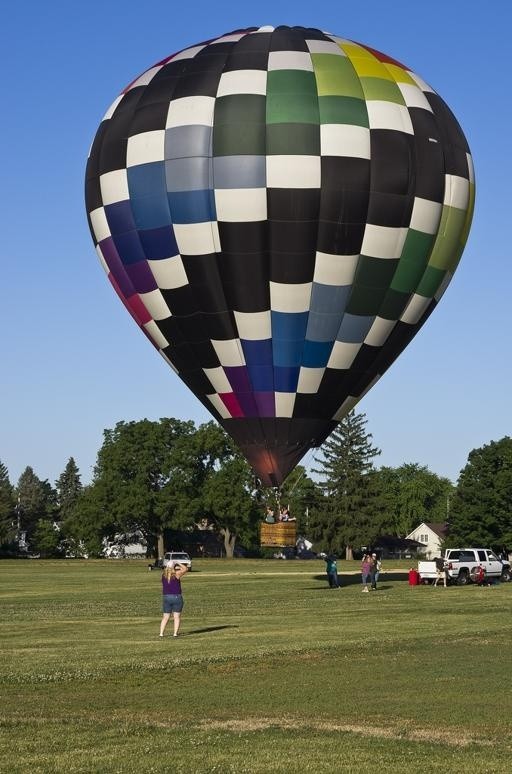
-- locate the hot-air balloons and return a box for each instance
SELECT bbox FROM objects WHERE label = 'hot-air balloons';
[86,26,476,546]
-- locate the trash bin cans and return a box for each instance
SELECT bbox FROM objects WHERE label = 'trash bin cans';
[409,571,418,585]
[149,564,152,571]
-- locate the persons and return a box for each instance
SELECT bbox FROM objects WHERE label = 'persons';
[264,505,297,524]
[324,549,341,589]
[433,552,448,587]
[361,552,374,593]
[158,560,189,639]
[369,553,381,590]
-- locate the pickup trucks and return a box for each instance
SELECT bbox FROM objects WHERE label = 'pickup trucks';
[418,548,512,586]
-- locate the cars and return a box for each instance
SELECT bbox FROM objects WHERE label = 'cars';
[160,552,192,571]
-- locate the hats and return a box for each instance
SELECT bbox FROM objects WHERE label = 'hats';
[167,560,176,568]
